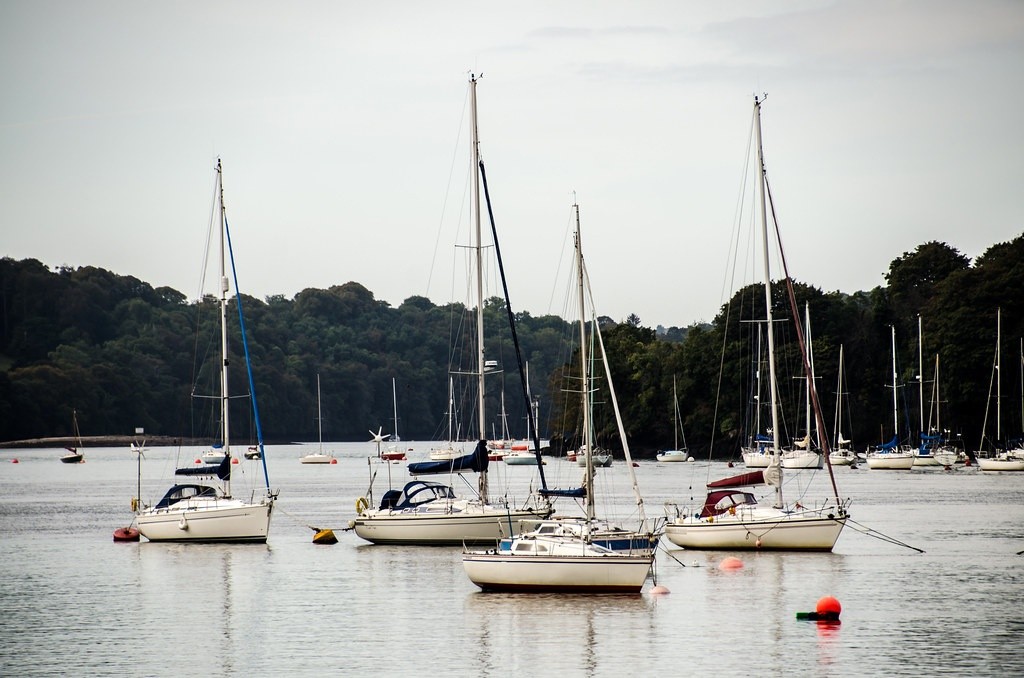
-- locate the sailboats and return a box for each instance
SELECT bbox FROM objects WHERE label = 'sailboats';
[173,309,262,476]
[59,409,86,463]
[657,84,855,551]
[741,299,1024,472]
[380,376,408,461]
[354,69,665,594]
[656,374,691,461]
[132,149,280,544]
[300,373,333,464]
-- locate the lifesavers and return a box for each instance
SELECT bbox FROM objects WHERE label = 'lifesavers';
[130,498,138,512]
[356,497,368,514]
